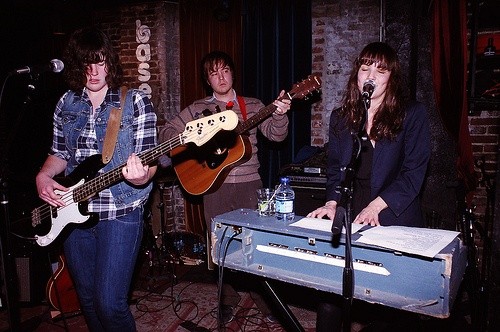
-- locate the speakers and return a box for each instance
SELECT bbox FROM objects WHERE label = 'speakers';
[294,192,331,220]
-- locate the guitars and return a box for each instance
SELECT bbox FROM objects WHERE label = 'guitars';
[173,73,321,196]
[29,100,239,247]
[47,254,79,314]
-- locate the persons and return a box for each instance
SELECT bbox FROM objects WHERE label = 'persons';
[306,43,431,332]
[36,28,158,332]
[155,51,291,322]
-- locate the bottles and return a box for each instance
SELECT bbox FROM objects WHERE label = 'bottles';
[273,177,295,222]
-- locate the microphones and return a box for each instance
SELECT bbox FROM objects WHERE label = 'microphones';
[13,58,65,74]
[362,80,376,101]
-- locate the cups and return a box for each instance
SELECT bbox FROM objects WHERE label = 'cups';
[256,187,275,218]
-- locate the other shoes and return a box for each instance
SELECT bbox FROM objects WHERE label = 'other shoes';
[217,305,235,322]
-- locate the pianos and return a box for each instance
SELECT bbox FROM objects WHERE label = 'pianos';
[210,208,469,332]
